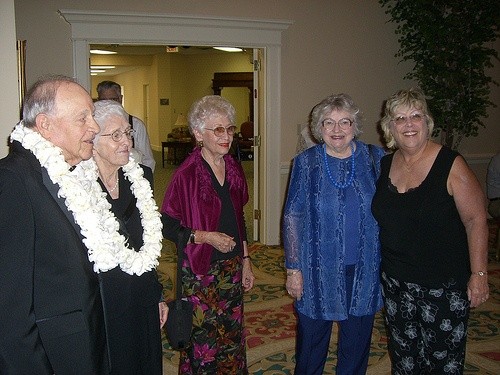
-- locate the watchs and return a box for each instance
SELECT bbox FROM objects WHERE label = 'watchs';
[471,270,487,276]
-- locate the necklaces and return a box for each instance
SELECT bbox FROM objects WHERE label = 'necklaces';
[323,142,355,188]
[7,120,127,272]
[83,153,163,276]
[109,178,119,192]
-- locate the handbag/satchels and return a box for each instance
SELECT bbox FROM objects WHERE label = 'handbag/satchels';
[164,296,193,352]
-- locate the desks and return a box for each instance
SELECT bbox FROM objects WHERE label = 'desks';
[162,141,194,168]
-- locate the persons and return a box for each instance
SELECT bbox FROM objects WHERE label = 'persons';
[370,86,488,375]
[160,95,254,375]
[0,73,111,375]
[282,94,388,375]
[71,99,170,375]
[96,80,156,180]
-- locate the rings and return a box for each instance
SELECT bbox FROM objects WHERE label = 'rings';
[482,298,486,303]
[288,290,291,295]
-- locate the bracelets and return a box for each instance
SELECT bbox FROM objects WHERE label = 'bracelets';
[243,255,251,260]
[190,230,196,244]
[286,270,296,275]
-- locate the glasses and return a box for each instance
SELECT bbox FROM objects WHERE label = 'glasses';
[204,126,237,137]
[101,129,136,142]
[321,118,354,130]
[390,114,426,125]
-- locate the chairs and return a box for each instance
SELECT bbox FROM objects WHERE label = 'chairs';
[237,121,254,161]
